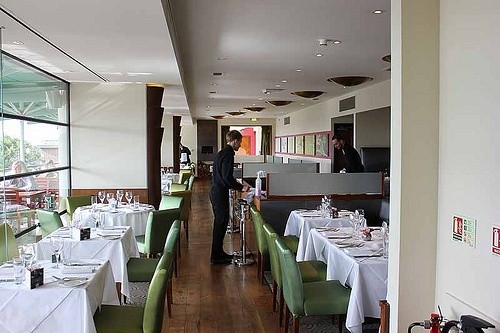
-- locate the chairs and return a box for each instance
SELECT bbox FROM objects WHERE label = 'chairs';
[93,169,352,333]
[36,209,64,239]
[66,195,91,222]
[0,223,20,267]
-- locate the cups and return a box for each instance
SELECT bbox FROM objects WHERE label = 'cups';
[98,213,105,227]
[91,196,97,207]
[134,196,139,209]
[109,199,115,211]
[14,258,24,284]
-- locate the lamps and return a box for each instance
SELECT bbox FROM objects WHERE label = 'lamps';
[209,76,370,119]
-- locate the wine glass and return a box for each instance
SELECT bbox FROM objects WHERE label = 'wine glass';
[97,190,132,208]
[349,209,366,240]
[50,236,64,270]
[73,213,82,230]
[91,208,100,231]
[19,245,34,273]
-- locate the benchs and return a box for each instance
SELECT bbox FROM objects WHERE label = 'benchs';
[234,155,320,191]
[0,187,35,234]
[30,189,60,223]
[246,172,385,271]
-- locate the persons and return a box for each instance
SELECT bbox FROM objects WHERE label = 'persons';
[209,130,250,264]
[3,161,38,190]
[180,143,191,165]
[332,134,365,173]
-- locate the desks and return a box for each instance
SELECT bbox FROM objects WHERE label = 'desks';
[0,153,187,333]
[284,209,390,333]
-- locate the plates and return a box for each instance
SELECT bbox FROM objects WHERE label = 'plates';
[57,277,87,287]
[0,267,26,281]
[313,226,381,248]
[295,209,353,219]
[63,258,102,267]
[102,234,120,240]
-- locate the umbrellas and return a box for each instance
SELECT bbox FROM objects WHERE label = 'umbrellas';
[0,71,59,162]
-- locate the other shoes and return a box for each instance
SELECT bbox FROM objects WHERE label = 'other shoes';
[224,252,234,258]
[210,258,232,264]
